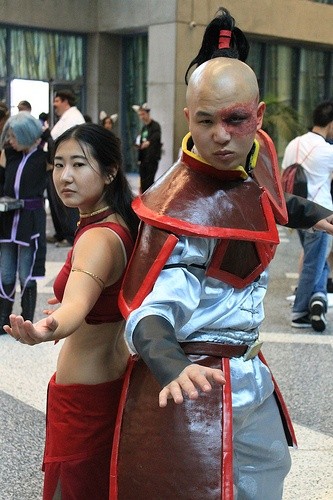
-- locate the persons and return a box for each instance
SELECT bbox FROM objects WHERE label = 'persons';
[0,91,161,334]
[4,123,143,500]
[117,9,333,500]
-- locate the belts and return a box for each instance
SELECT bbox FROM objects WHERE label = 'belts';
[0,199,47,212]
[179,338,264,360]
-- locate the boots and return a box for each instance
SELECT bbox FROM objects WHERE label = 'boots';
[0,283,17,336]
[21,279,37,324]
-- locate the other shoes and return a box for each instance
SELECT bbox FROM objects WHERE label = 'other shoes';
[56,238,75,248]
[309,299,328,332]
[327,278,333,293]
[45,236,59,243]
[291,313,313,327]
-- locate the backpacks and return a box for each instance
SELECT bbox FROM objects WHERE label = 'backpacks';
[280,136,323,199]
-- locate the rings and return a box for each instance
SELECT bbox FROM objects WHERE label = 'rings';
[16,338,21,341]
